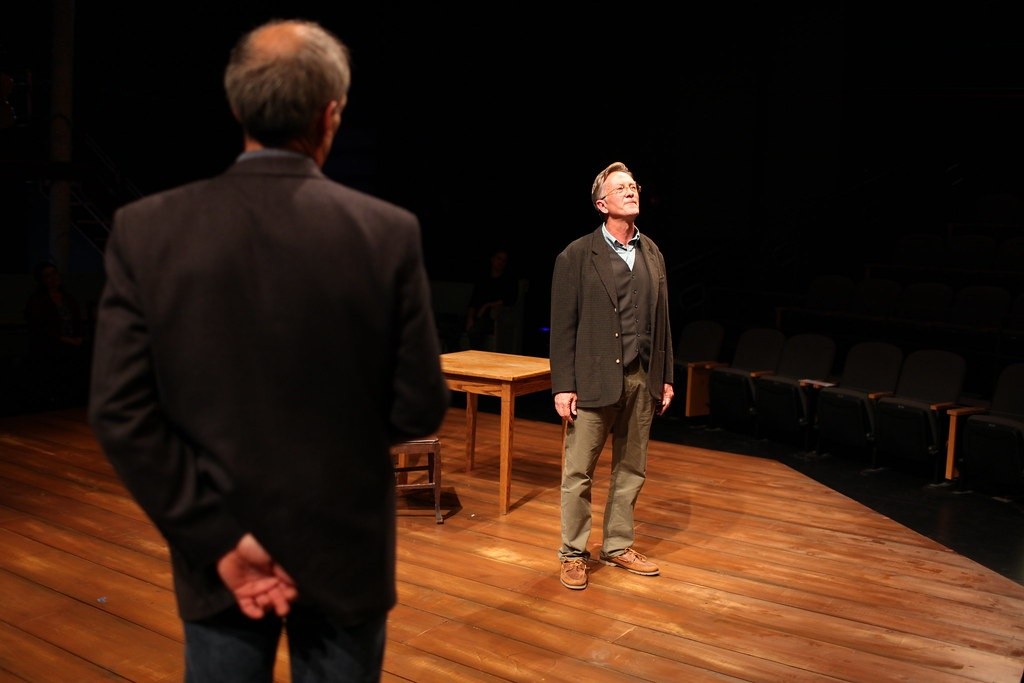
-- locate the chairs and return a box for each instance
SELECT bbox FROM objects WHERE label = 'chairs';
[667,323,1024,500]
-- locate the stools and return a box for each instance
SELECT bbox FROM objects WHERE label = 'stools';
[390,433,444,523]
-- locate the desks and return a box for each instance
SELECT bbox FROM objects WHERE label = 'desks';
[398,350,569,514]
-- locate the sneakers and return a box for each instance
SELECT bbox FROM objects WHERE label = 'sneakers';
[560,554,589,591]
[599,547,660,576]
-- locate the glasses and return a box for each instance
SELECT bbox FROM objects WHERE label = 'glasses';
[595,184,642,206]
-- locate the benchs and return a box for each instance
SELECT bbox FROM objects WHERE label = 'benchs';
[428,281,522,355]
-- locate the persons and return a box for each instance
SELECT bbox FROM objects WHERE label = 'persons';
[24,260,86,413]
[550,162,674,589]
[89,22,448,683]
[460,249,517,349]
